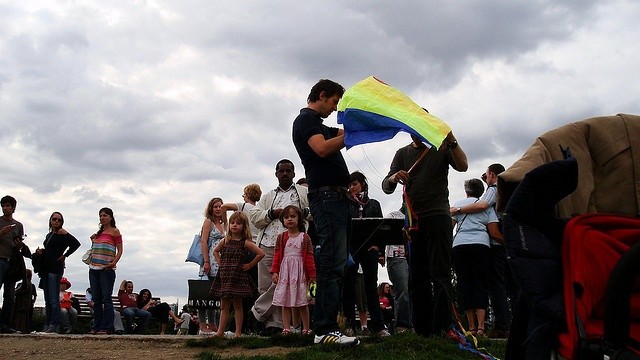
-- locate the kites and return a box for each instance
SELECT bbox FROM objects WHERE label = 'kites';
[337,76,460,154]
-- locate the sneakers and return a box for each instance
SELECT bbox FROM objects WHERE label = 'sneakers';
[199,329,217,336]
[314,331,361,346]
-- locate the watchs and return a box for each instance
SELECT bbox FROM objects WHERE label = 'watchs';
[450,139,457,149]
[457,206,461,213]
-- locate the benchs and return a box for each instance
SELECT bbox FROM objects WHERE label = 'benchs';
[73,290,164,332]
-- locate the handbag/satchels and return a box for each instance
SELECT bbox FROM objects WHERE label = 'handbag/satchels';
[185,234,211,264]
[188,279,221,308]
[83,249,94,264]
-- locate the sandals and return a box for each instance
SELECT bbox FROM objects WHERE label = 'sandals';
[301,329,313,335]
[281,329,293,335]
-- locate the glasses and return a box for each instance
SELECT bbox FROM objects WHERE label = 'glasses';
[53,218,62,222]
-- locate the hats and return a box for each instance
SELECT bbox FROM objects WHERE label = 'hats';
[60,277,71,290]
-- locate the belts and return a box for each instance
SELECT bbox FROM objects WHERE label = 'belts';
[310,186,348,193]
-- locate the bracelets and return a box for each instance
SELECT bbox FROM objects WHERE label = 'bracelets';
[204,260,210,263]
[19,246,23,250]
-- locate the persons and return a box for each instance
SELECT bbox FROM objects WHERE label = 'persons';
[220,183,262,263]
[342,172,388,337]
[452,179,504,337]
[190,310,199,335]
[379,283,394,328]
[0,195,24,334]
[86,288,126,335]
[118,280,151,333]
[270,205,317,335]
[296,178,308,186]
[30,212,81,336]
[59,277,77,334]
[137,289,185,335]
[210,211,265,339]
[382,108,468,337]
[14,269,37,332]
[387,192,415,334]
[355,263,369,331]
[248,159,313,339]
[449,164,510,338]
[89,208,123,334]
[197,198,225,336]
[176,306,193,335]
